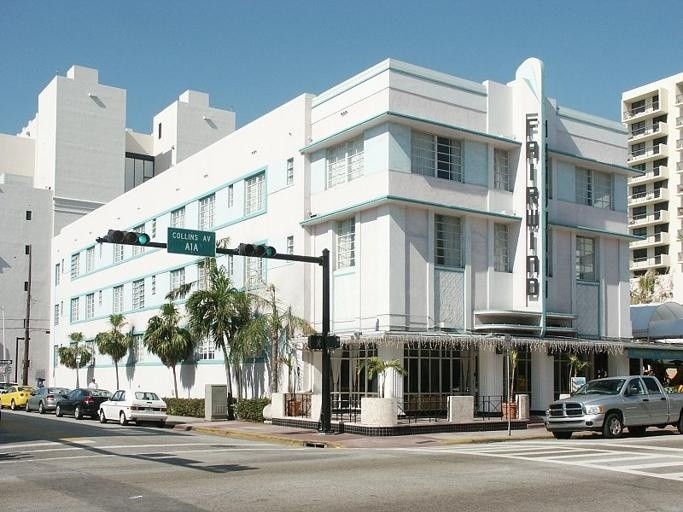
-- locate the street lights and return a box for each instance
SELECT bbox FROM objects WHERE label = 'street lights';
[14,337,30,383]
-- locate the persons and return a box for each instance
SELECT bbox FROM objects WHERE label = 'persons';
[88,378,97,389]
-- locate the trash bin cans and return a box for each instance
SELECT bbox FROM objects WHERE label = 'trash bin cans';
[515,394,531,421]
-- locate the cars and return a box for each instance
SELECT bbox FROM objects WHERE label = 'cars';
[0,381,167,426]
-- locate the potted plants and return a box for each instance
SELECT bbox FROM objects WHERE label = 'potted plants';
[502,347,519,418]
[353,355,408,425]
[279,357,300,416]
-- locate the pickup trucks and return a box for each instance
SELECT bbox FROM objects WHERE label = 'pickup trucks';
[544,373,682,438]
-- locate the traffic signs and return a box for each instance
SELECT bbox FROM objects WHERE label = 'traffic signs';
[166,227,216,257]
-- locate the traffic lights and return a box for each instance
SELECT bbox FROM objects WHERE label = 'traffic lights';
[107,230,150,246]
[237,243,276,259]
